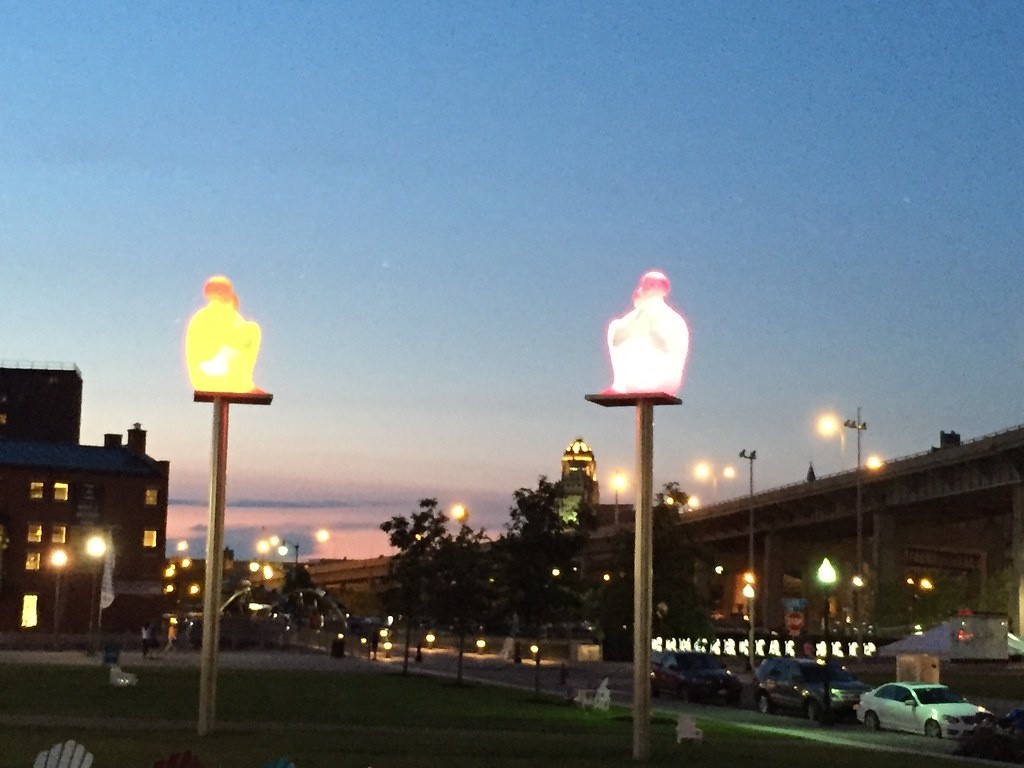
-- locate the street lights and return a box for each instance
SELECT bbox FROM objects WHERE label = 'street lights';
[84,534,105,655]
[49,548,68,648]
[278,539,299,568]
[184,275,274,738]
[739,449,757,667]
[584,270,691,763]
[817,555,837,657]
[845,407,866,655]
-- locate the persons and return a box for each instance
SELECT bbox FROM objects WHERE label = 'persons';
[142,621,151,658]
[368,625,379,662]
[164,623,178,654]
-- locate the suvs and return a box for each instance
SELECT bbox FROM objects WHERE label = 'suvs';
[752,657,874,723]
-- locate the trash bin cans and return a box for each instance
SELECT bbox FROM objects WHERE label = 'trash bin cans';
[104,643,118,662]
[572,643,600,662]
[331,639,345,658]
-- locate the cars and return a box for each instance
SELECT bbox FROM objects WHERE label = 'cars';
[1006,708,1024,733]
[855,681,999,741]
[649,652,742,705]
